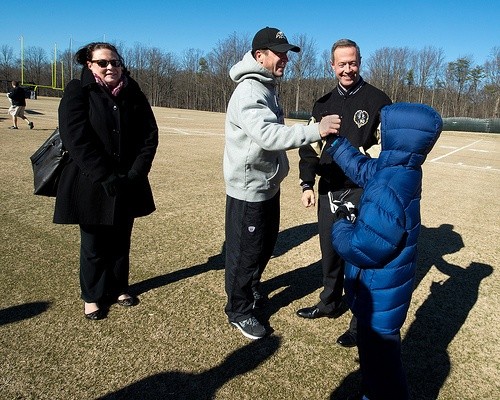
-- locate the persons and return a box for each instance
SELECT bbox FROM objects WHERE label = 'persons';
[329,103,443,400]
[296,40,393,347]
[53,42,159,320]
[9,81,33,129]
[223,27,341,339]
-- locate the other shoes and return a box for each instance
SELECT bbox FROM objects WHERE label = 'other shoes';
[13,126,18,130]
[116,294,133,306]
[84,302,100,319]
[28,122,34,129]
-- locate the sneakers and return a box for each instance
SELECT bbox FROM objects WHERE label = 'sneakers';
[252,287,270,309]
[231,318,267,340]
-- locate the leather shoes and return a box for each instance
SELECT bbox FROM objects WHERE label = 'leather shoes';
[337,328,358,348]
[296,305,337,319]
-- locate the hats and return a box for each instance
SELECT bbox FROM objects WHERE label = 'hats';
[250,26,301,53]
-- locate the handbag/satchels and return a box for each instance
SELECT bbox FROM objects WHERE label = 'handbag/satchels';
[30,128,68,197]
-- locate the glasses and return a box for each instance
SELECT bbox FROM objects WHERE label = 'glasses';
[90,58,122,68]
